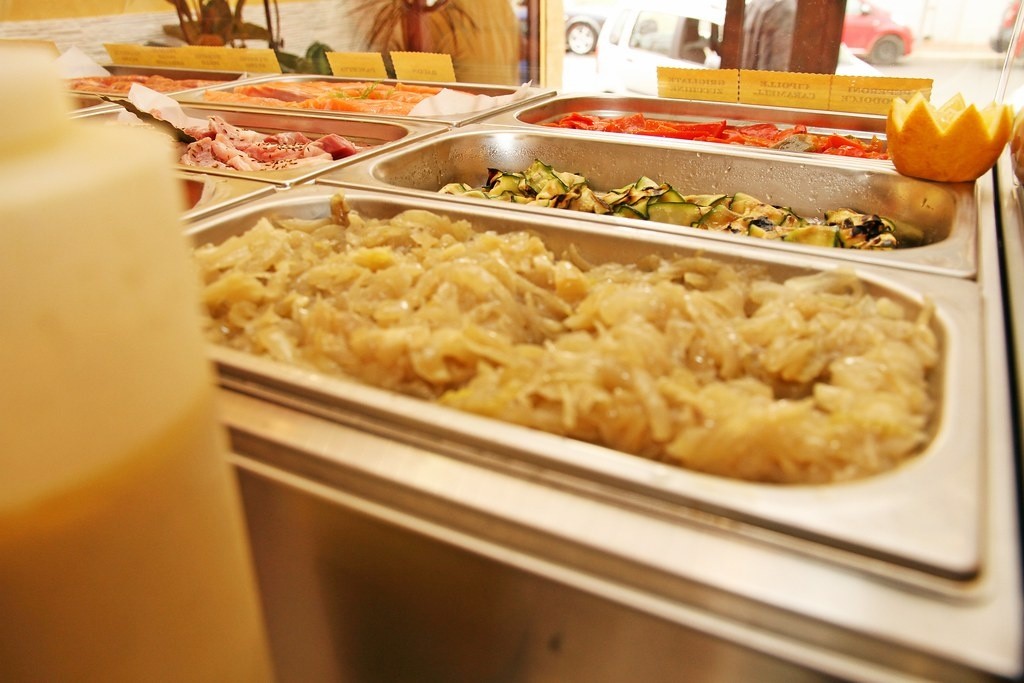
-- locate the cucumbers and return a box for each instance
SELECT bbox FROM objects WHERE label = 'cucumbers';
[438,157,897,252]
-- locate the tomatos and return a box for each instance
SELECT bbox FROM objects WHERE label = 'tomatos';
[541,112,892,161]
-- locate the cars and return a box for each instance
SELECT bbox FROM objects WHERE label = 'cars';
[564,14,604,57]
[842,3,914,66]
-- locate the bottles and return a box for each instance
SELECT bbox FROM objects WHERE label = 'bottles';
[0,42,279,683]
